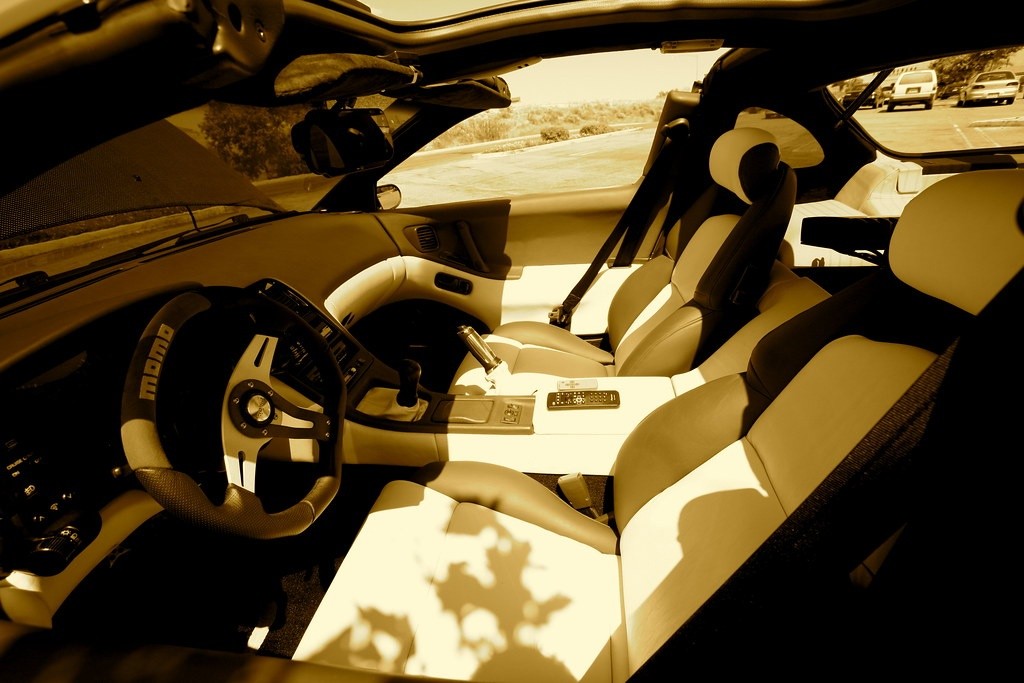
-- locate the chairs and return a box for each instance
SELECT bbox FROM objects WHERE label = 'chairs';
[451,105,824,395]
[283,168,1024,683]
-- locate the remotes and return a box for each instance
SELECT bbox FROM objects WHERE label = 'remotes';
[558,378,598,391]
[547,389,619,410]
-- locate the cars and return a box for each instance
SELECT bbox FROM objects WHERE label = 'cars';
[958,70,1019,105]
[841,82,883,112]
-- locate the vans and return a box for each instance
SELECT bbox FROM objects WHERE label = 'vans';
[887,69,938,112]
